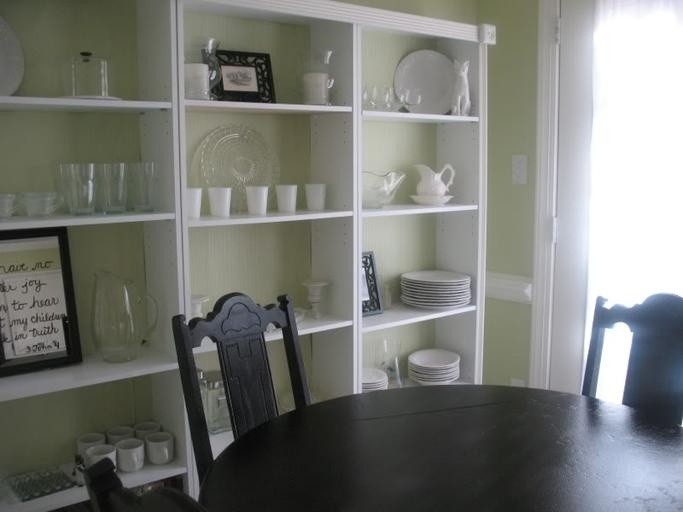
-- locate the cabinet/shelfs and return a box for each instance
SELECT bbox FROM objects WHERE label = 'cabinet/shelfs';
[0,2,499,512]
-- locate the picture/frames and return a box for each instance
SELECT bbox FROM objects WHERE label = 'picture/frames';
[202,44,278,104]
[355,245,385,317]
[0,226,86,378]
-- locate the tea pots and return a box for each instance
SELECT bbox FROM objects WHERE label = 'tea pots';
[412,161,456,195]
[88,268,159,362]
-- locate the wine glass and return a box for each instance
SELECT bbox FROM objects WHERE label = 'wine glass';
[190,294,208,318]
[300,279,328,321]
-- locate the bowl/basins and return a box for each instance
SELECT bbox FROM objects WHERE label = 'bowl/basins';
[360,169,406,210]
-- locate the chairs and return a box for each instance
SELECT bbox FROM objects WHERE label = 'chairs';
[164,288,315,492]
[79,459,202,510]
[583,293,682,434]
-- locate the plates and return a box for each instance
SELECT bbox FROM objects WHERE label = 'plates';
[393,49,458,114]
[64,93,122,103]
[361,367,387,394]
[398,269,471,311]
[0,16,26,95]
[408,194,455,206]
[197,126,281,208]
[407,348,459,385]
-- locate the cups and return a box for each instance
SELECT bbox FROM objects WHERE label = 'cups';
[57,160,154,215]
[0,189,64,221]
[292,47,333,106]
[183,36,220,101]
[360,82,423,113]
[186,183,326,217]
[74,419,176,479]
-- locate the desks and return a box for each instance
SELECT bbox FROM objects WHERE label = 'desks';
[198,383,683,512]
